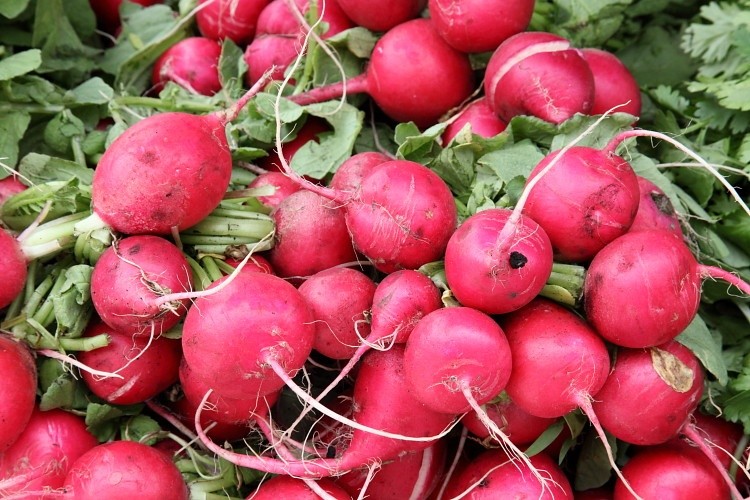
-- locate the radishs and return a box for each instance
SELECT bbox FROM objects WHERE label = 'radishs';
[0,0,750,500]
